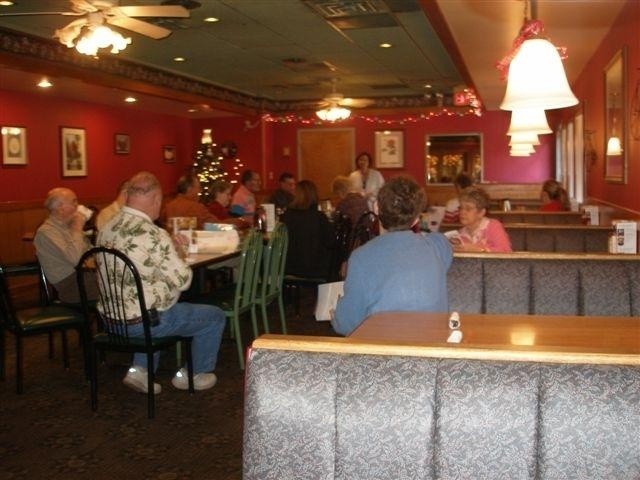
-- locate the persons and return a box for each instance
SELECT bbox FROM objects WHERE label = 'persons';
[96,170,228,396]
[268,173,297,209]
[163,174,221,231]
[449,185,513,253]
[327,175,376,255]
[32,187,132,366]
[535,179,571,212]
[329,173,453,337]
[229,168,263,216]
[348,151,388,223]
[279,179,341,281]
[442,174,474,225]
[94,179,132,231]
[205,180,256,230]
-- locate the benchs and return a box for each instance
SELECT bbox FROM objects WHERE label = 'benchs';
[243,198,640,480]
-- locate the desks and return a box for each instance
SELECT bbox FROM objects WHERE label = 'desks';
[82,224,241,375]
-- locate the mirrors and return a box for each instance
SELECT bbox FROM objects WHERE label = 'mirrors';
[424,133,484,187]
[602,45,628,185]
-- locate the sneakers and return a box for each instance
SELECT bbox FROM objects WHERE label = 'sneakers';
[171,367,218,391]
[124,365,162,395]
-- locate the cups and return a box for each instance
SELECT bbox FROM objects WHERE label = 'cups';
[76,204,93,221]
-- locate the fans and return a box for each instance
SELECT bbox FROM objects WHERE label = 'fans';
[308,77,374,108]
[0,0,191,40]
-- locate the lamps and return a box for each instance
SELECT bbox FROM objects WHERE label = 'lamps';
[499,2,580,157]
[606,94,622,157]
[75,27,132,59]
[315,104,351,123]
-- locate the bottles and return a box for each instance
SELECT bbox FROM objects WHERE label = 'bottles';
[586,211,591,225]
[608,232,617,254]
[503,199,511,212]
[254,206,264,232]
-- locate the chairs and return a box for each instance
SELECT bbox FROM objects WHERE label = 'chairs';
[34,222,72,372]
[208,228,264,373]
[0,259,91,395]
[229,222,289,337]
[74,247,196,419]
[289,211,378,318]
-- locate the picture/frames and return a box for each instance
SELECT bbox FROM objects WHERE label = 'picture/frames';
[114,133,130,155]
[60,126,88,177]
[370,126,406,170]
[2,124,28,167]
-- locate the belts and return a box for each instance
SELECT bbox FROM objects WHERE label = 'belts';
[102,314,143,325]
